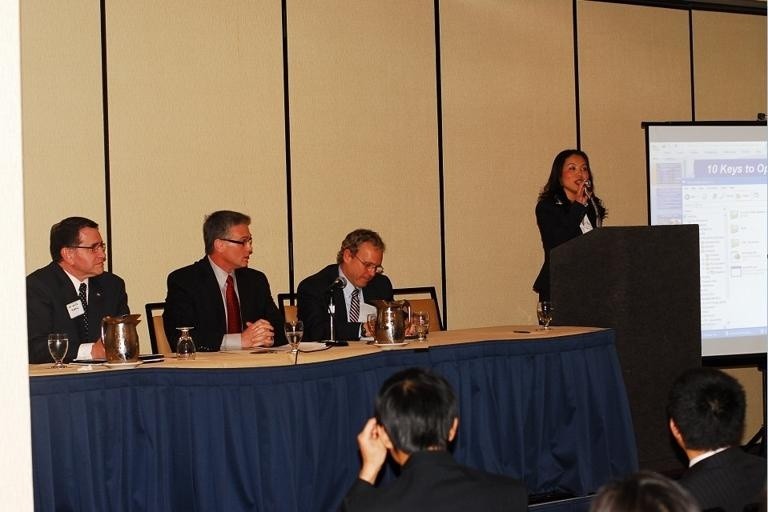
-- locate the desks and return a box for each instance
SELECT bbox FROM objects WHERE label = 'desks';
[28,323,641,512]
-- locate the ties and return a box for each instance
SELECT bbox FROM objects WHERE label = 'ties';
[348,287,360,323]
[225,274,243,335]
[77,284,91,343]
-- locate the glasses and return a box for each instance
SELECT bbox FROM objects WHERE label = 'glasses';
[354,254,384,276]
[218,238,254,247]
[64,243,108,254]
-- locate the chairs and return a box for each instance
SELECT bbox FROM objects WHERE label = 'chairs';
[277,292,299,323]
[144,302,174,355]
[391,286,443,332]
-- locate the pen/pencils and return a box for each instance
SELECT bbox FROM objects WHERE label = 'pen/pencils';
[514,329,531,335]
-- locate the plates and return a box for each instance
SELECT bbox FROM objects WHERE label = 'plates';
[102,362,143,369]
[373,343,408,346]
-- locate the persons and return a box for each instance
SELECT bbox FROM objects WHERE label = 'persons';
[26,217,130,364]
[162,211,289,351]
[533,149,605,325]
[591,470,702,512]
[337,368,528,512]
[667,367,768,512]
[296,229,394,341]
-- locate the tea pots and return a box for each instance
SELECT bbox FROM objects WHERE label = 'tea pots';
[372,299,411,343]
[100,314,141,363]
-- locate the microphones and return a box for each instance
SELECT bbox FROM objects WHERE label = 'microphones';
[325,278,348,298]
[583,180,591,192]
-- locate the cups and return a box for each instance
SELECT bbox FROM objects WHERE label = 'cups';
[368,314,378,343]
[537,302,555,330]
[284,322,304,354]
[48,333,69,368]
[413,311,431,343]
[176,327,196,360]
